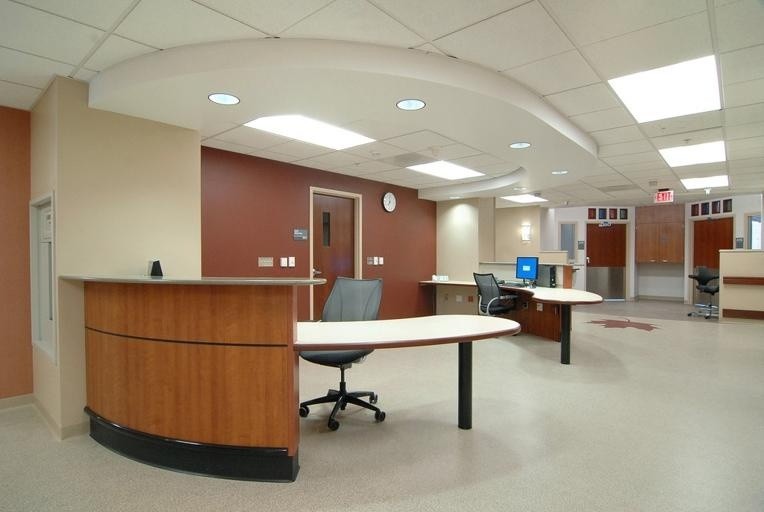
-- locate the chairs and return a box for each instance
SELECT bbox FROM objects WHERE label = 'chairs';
[687,265,718,319]
[472,273,519,336]
[296,277,387,431]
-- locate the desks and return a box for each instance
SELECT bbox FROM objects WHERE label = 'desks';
[419,261,604,365]
[718,249,764,325]
[63,275,520,482]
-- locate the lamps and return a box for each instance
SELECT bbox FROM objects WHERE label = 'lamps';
[519,224,532,241]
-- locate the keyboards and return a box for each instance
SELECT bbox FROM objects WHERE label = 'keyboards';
[502,282,523,288]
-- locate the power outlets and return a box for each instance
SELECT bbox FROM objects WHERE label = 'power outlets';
[522,302,529,310]
[536,303,543,312]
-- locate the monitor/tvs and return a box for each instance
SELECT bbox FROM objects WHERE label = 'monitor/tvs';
[516,256,538,286]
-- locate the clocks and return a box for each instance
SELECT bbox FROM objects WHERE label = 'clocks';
[381,191,397,213]
